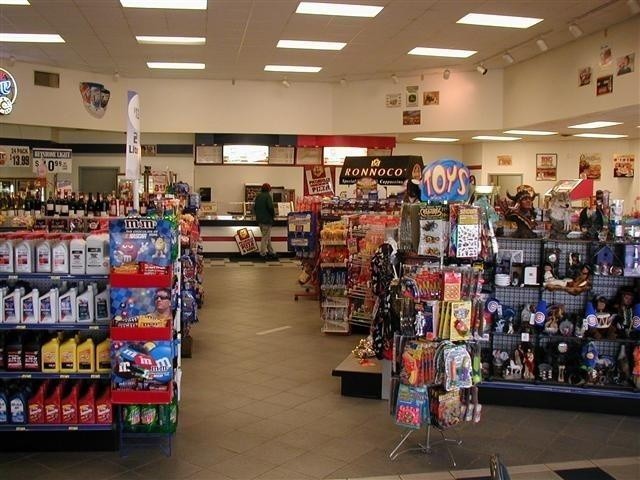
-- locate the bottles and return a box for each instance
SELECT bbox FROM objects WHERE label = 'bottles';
[0,189,174,217]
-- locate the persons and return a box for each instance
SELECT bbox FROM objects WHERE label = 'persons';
[579,200,603,240]
[254,183,278,262]
[155,289,170,318]
[617,55,631,76]
[506,190,540,238]
[395,254,640,426]
[394,253,639,426]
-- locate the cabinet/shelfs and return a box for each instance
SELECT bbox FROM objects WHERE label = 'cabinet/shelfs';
[0,216,115,452]
[381,231,639,400]
[348,211,402,326]
[320,237,349,334]
[109,216,182,457]
